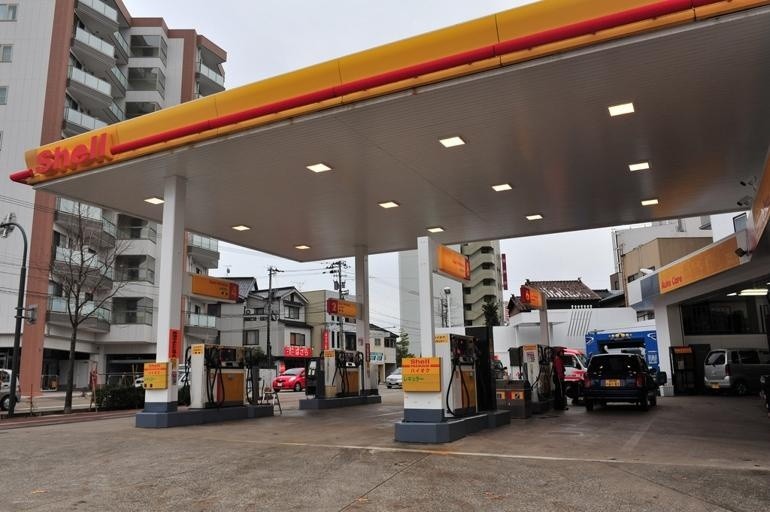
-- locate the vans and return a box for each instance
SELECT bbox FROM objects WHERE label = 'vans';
[703,346,770,396]
[560,348,590,398]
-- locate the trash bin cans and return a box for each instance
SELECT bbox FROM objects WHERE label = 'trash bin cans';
[495,380,533,419]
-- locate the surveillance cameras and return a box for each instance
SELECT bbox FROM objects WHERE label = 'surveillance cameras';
[737,195,755,206]
[739,174,758,191]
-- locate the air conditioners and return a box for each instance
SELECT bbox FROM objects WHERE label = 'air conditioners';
[245,307,254,315]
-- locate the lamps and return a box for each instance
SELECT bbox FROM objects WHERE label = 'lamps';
[734,247,749,257]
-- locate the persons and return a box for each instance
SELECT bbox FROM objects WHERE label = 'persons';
[553,350,569,411]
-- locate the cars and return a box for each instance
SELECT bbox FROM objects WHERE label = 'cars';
[386,366,402,389]
[582,352,659,412]
[273,367,306,393]
[493,359,508,380]
[136,363,191,393]
[1,368,21,409]
